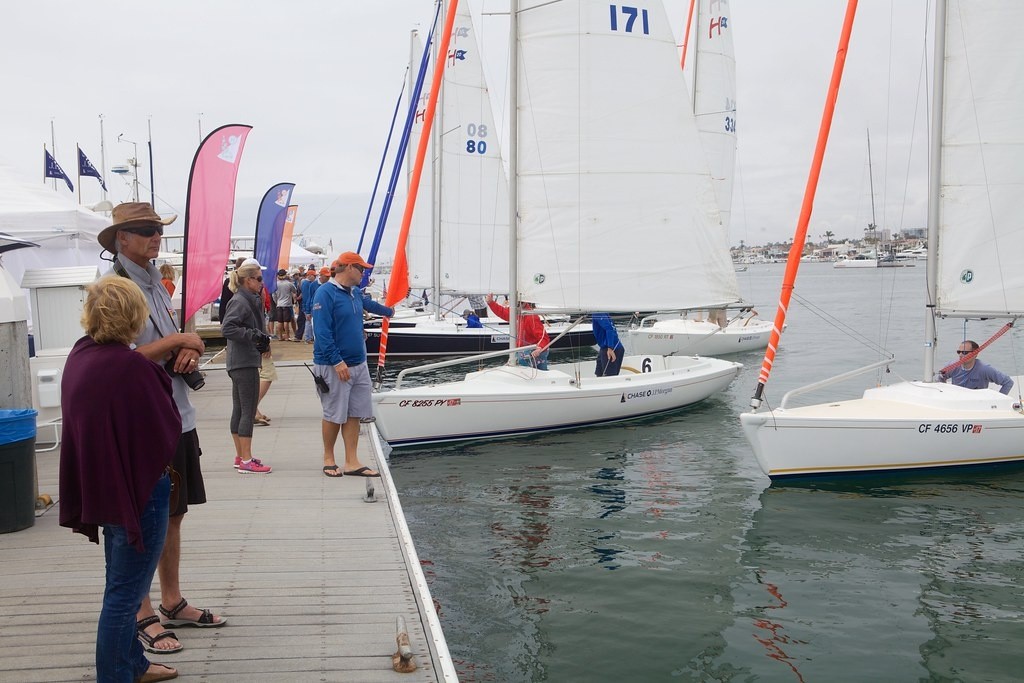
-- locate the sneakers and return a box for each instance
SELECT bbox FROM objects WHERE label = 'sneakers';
[234,456,262,467]
[237,459,272,473]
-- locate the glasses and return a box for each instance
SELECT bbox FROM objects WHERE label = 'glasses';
[957,350,973,355]
[249,276,263,282]
[118,224,164,237]
[353,264,365,273]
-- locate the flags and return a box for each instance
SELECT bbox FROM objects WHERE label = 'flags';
[423,290,429,305]
[45,150,74,193]
[76,146,108,192]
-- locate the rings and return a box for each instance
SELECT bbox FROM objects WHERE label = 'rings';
[191,358,195,362]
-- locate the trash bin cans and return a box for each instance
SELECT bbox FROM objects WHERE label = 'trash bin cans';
[0,409,38,534]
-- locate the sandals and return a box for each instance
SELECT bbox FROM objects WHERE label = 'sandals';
[159,596,229,628]
[135,616,184,654]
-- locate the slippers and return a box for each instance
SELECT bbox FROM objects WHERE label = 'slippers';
[323,464,343,477]
[343,467,381,477]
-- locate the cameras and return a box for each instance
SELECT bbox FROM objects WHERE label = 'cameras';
[180,360,206,391]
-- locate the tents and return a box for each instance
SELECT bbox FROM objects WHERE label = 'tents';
[142,246,184,276]
[0,184,113,337]
[250,237,328,267]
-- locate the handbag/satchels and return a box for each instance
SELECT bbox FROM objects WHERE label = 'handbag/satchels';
[164,466,181,515]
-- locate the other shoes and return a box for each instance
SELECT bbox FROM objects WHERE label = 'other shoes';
[360,416,376,422]
[134,663,179,683]
[267,335,315,344]
[253,412,272,425]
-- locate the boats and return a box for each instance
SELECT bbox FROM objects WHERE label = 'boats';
[800,242,927,267]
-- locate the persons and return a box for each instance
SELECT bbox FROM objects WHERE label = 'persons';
[95,202,227,655]
[259,263,331,344]
[484,292,551,370]
[59,276,183,683]
[590,310,625,377]
[158,262,175,297]
[220,255,279,474]
[310,251,395,478]
[934,341,1016,396]
[462,309,484,329]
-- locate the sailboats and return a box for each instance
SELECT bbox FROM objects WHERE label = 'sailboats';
[738,0,1024,479]
[360,0,787,452]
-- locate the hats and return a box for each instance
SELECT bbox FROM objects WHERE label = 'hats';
[320,266,331,277]
[241,258,268,270]
[291,268,301,276]
[279,269,288,276]
[97,203,179,254]
[337,252,374,269]
[331,260,339,268]
[308,270,319,276]
